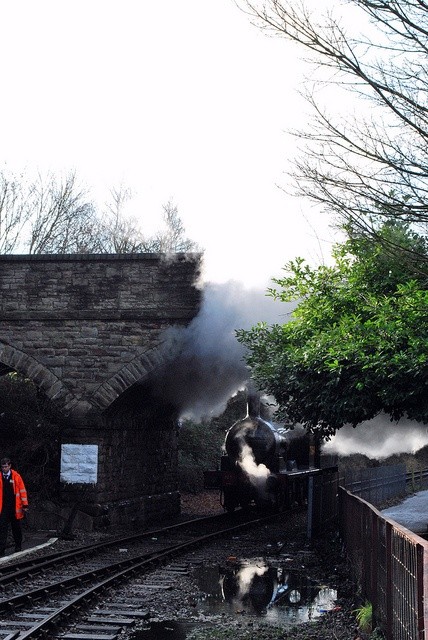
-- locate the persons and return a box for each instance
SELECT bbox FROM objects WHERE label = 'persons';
[1,459,30,552]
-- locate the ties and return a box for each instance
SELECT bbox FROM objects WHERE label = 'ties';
[4,475,8,478]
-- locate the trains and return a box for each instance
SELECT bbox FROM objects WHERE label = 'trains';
[219,393,323,513]
